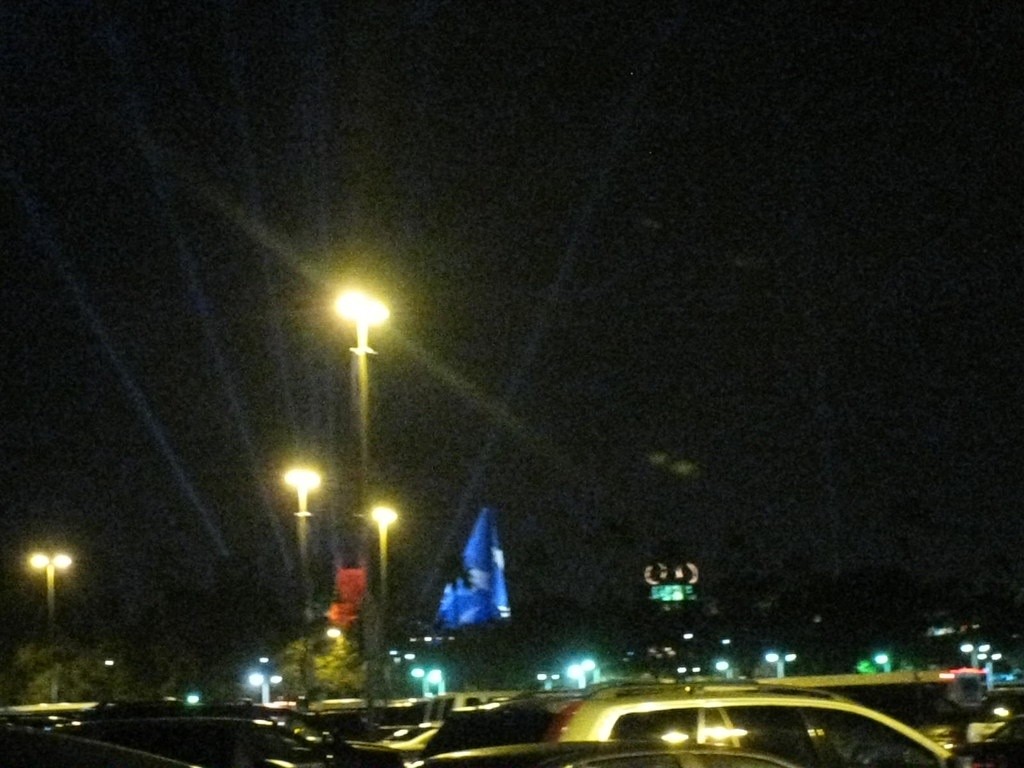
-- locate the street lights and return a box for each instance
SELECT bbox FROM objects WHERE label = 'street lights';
[875,655,890,673]
[283,462,323,708]
[977,652,1002,691]
[366,501,401,675]
[567,661,596,689]
[766,652,797,678]
[26,550,75,702]
[959,642,992,671]
[714,660,728,682]
[334,293,389,575]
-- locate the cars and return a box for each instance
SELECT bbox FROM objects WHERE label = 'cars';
[0,670,1024,768]
